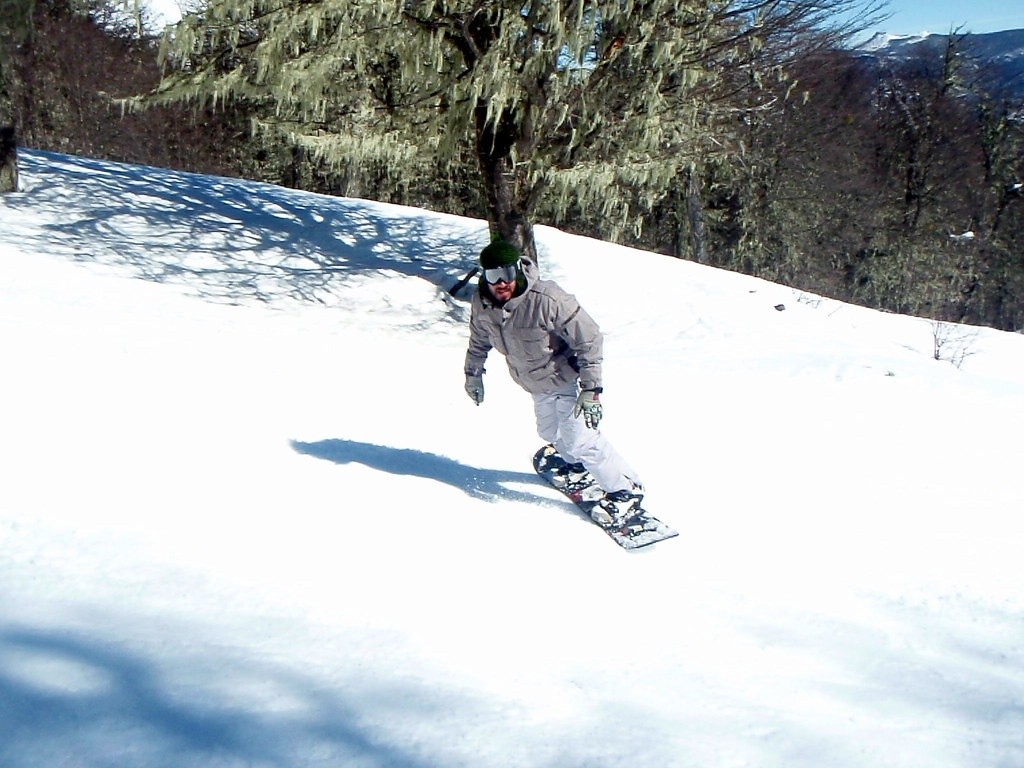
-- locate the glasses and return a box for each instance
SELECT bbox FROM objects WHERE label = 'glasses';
[483,264,519,285]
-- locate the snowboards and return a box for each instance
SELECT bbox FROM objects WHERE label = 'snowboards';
[532,443,680,550]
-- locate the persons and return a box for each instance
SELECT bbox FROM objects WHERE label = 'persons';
[464,240,643,526]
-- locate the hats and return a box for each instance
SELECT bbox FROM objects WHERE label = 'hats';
[479,241,520,270]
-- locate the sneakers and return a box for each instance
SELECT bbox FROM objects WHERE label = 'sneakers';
[550,463,590,488]
[590,479,647,525]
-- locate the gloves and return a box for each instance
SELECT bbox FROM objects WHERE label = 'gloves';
[572,390,604,431]
[464,373,484,408]
